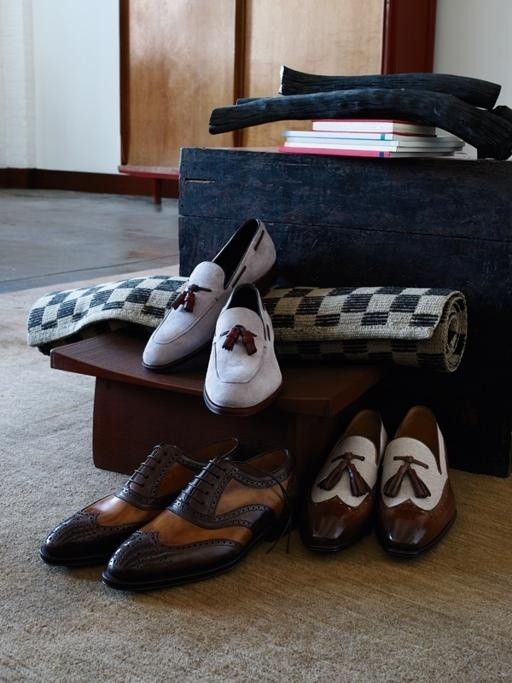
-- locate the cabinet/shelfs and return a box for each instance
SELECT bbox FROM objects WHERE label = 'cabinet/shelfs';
[118,0,436,206]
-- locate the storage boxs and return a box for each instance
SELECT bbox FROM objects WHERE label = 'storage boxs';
[179,147,511,480]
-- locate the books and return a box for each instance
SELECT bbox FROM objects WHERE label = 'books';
[276,117,465,158]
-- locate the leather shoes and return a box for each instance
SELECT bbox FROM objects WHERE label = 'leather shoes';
[102,438,295,591]
[39,436,240,567]
[204,284,285,416]
[377,406,457,558]
[143,218,277,371]
[300,410,387,553]
